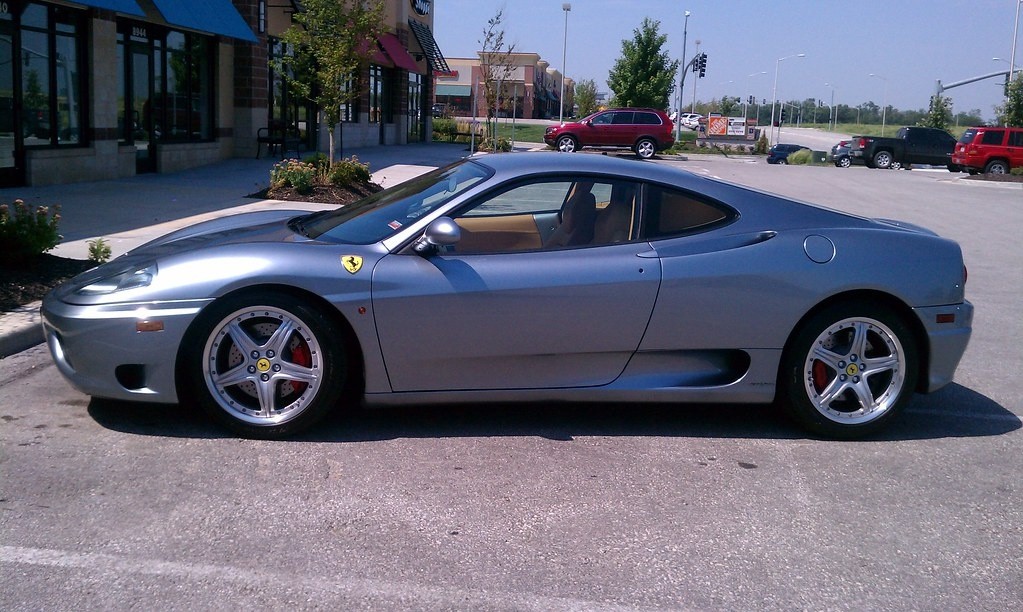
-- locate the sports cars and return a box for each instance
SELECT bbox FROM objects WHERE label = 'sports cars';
[42,152,975,434]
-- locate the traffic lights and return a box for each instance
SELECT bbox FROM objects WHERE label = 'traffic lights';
[698,55,707,77]
[749,95,752,104]
[1004,73,1011,95]
[693,55,698,72]
[762,98,766,105]
[781,108,785,120]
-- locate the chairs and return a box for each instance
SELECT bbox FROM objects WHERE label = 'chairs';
[589,200,630,245]
[543,191,597,249]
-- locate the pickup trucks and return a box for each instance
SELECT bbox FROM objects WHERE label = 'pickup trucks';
[0,96,63,140]
[854,127,960,168]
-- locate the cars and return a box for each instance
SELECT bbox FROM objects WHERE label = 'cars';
[768,143,812,165]
[430,104,449,118]
[669,112,678,123]
[831,139,855,167]
[952,128,1023,174]
[682,115,702,124]
[689,119,699,131]
[142,94,201,132]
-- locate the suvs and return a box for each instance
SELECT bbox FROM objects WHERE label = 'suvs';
[543,109,673,157]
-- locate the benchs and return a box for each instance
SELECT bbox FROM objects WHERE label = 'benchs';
[255,124,301,161]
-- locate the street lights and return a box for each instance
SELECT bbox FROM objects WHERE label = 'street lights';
[870,73,887,137]
[825,81,838,129]
[682,11,690,75]
[560,3,571,127]
[769,54,805,149]
[745,72,768,131]
